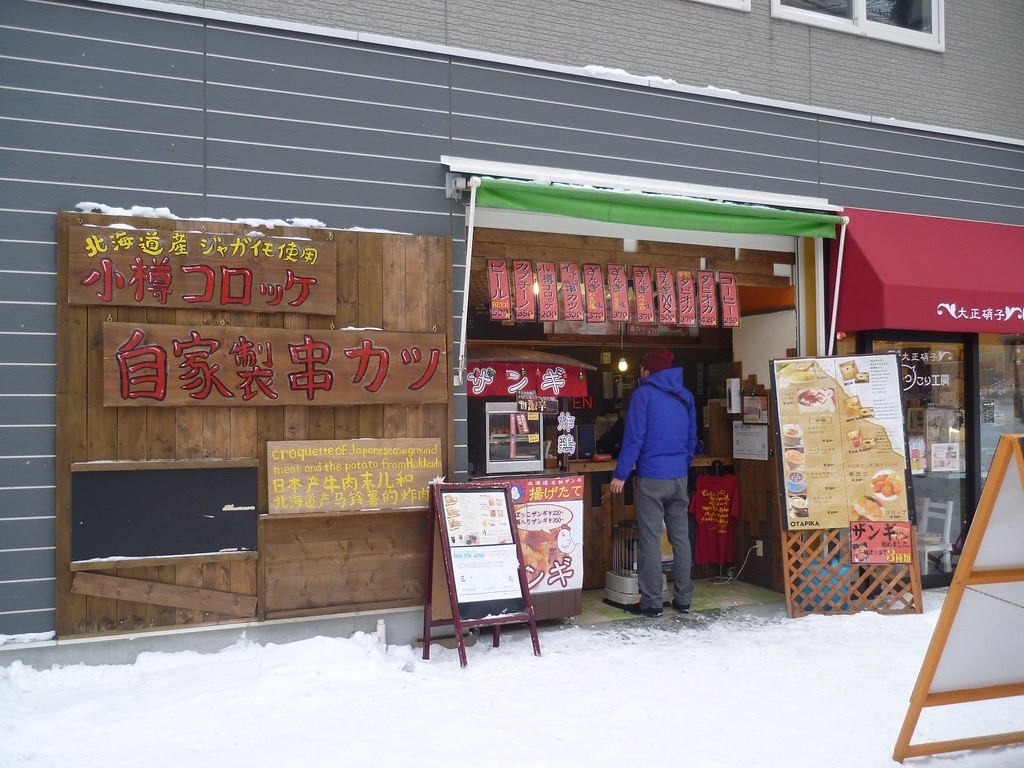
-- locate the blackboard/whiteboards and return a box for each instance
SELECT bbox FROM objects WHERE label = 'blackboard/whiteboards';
[431,482,535,628]
[70,458,262,570]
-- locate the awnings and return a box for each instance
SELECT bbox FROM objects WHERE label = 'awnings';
[827,204,1024,335]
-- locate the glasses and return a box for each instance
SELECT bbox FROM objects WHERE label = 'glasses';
[639,364,644,368]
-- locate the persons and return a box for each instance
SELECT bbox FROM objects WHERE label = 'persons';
[611,346,697,617]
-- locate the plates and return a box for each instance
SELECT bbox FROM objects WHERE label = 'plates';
[871,470,903,501]
[854,496,884,521]
[781,375,816,383]
[788,483,807,493]
[797,388,829,408]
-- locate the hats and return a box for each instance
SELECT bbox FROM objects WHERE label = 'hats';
[643,349,674,375]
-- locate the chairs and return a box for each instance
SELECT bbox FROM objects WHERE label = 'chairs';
[916,499,954,575]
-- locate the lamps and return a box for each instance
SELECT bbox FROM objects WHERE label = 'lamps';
[951,411,965,433]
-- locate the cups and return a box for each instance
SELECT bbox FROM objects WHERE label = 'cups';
[790,497,806,513]
[787,472,807,493]
[783,425,803,445]
[841,363,855,378]
[847,430,862,448]
[785,450,803,470]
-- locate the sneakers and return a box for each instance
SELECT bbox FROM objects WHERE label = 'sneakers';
[625,603,663,618]
[672,599,690,613]
